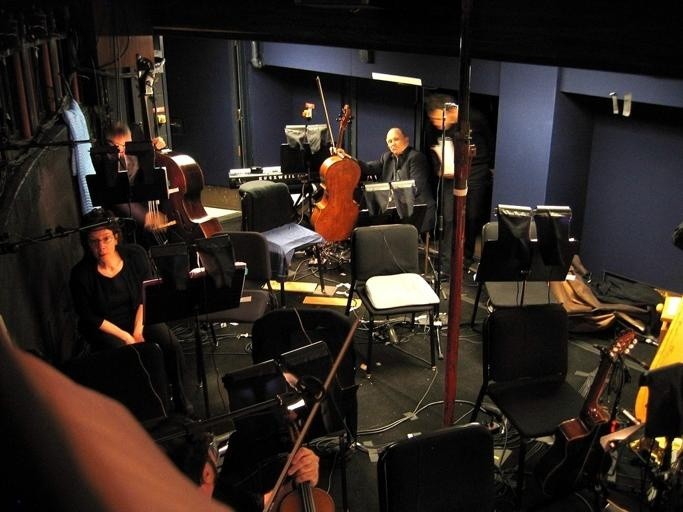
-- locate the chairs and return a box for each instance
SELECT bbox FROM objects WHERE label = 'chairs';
[246,311,361,509]
[234,180,328,306]
[469,201,581,330]
[194,229,273,355]
[425,148,445,250]
[342,222,441,373]
[374,420,502,512]
[470,303,588,504]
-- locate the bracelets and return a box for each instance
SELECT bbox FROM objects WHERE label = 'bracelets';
[290,479,298,491]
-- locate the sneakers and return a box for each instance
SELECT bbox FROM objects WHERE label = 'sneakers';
[463,258,472,270]
[435,272,448,282]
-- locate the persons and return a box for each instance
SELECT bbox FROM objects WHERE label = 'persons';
[99,121,169,233]
[66,206,195,422]
[424,94,485,284]
[146,410,324,511]
[328,126,436,236]
[212,373,300,511]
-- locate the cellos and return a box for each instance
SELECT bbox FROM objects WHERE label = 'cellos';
[274,396,335,511]
[135,54,224,269]
[531,328,637,510]
[311,104,360,243]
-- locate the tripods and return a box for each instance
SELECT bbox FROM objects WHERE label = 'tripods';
[402,106,471,360]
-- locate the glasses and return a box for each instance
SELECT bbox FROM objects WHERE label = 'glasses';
[88,236,113,244]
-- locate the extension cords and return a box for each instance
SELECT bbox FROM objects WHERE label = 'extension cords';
[486,421,500,434]
[308,258,318,267]
